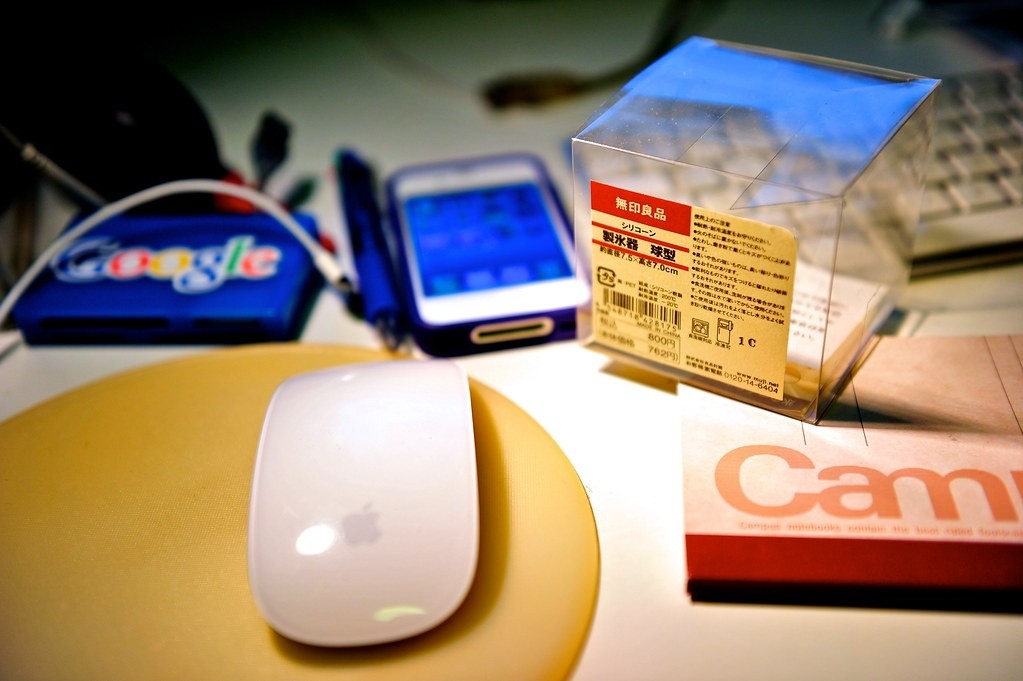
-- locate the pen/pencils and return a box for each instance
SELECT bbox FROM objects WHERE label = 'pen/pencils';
[331,146,404,353]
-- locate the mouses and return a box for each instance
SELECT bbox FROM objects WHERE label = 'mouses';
[247,357,480,646]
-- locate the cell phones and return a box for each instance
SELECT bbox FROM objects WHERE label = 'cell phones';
[384,150,592,359]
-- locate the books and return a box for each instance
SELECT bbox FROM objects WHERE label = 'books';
[676,332,1023,587]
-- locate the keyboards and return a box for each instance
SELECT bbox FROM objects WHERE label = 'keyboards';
[584,57,1023,279]
